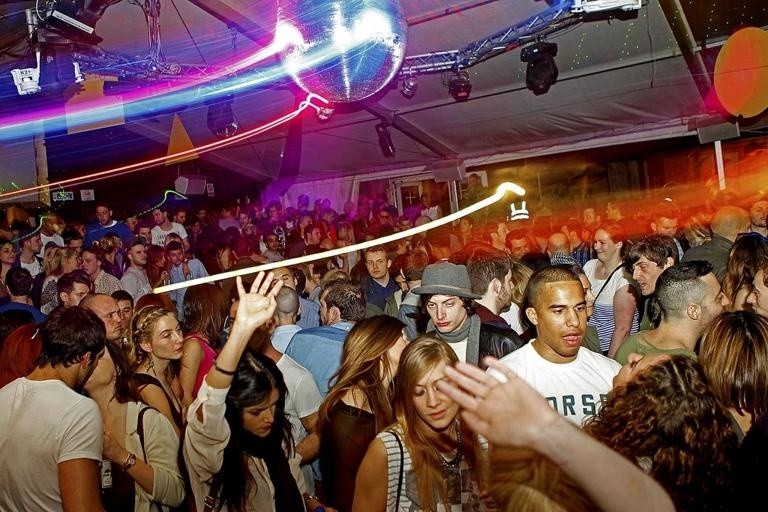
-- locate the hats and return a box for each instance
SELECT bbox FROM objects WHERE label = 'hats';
[411,264,482,301]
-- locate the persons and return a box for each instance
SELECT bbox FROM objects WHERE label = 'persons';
[2,173,768,511]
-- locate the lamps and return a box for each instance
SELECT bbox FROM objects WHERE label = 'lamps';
[521,36,558,95]
[448,66,472,102]
[401,71,418,100]
[205,96,237,139]
[375,123,395,158]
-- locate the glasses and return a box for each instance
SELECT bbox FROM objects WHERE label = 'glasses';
[380,215,389,219]
